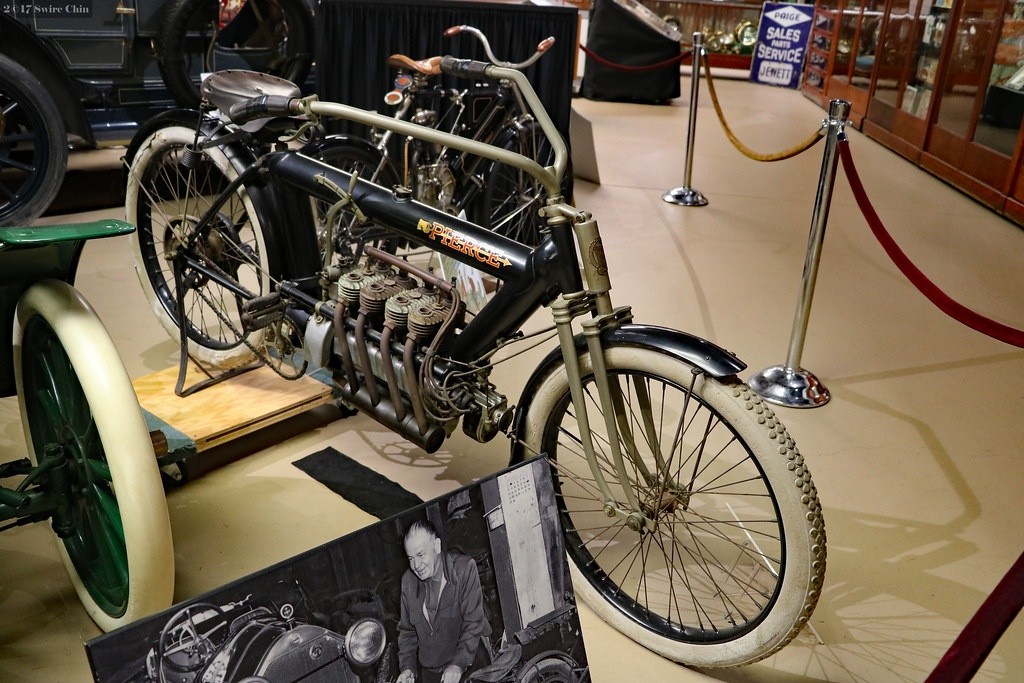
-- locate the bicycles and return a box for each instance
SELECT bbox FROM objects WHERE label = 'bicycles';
[279,23,575,279]
[0,3,317,224]
[122,49,827,672]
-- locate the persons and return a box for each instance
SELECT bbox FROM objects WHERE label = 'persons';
[395,519,491,683]
